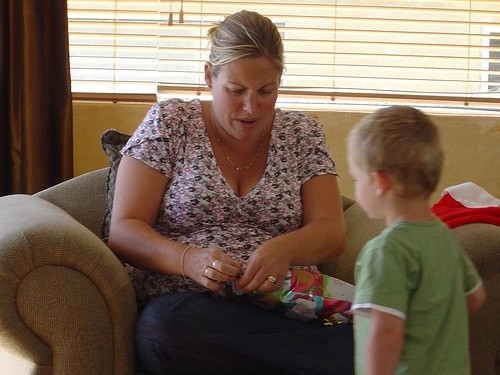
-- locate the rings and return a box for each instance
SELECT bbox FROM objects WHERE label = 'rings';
[267,276,276,283]
[205,268,211,276]
[213,260,219,270]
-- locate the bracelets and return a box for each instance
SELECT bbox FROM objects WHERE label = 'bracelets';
[180,245,193,284]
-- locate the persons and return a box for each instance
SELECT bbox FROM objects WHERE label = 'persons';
[348,105,486,375]
[109,10,354,375]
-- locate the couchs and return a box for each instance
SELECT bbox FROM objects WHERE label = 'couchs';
[0,166,500,375]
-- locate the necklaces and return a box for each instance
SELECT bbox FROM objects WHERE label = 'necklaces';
[209,102,267,172]
